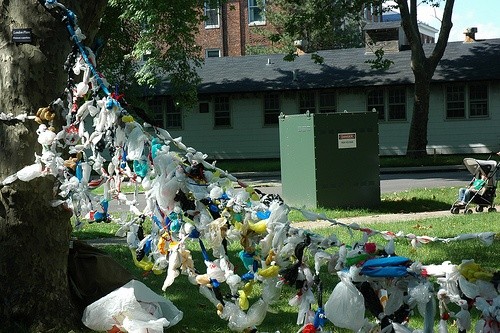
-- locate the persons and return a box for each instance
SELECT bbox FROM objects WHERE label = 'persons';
[453,172,489,205]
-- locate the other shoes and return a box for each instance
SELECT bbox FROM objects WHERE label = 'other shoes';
[458,200,467,205]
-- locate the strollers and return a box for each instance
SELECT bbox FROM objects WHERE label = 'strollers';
[450,152,500,214]
